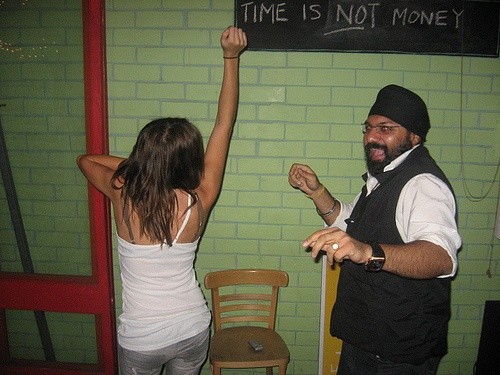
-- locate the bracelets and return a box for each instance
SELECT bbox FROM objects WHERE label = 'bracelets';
[223,56,239,59]
[305,184,325,199]
[316,199,336,216]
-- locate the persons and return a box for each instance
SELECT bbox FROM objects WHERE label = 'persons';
[288,84,461,375]
[77,25,248,375]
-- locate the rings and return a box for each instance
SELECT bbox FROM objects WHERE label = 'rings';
[331,242,339,250]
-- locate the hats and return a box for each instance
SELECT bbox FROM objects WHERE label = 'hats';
[367,84,431,141]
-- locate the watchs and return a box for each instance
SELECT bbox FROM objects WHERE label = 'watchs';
[363,241,387,271]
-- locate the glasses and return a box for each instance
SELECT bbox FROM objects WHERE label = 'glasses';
[360,123,403,133]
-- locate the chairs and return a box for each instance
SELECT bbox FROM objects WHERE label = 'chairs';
[204,268,290,375]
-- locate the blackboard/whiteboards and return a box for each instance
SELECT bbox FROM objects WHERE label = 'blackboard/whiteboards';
[233,0,500,58]
